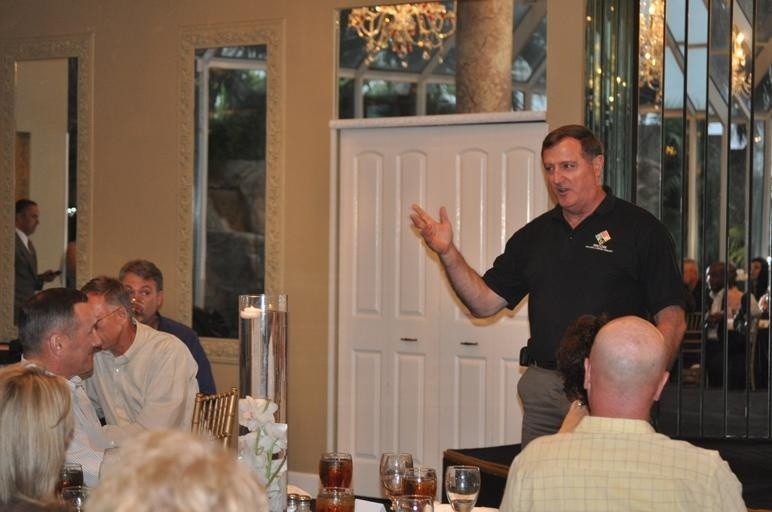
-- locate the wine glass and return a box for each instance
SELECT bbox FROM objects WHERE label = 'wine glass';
[380,452,414,510]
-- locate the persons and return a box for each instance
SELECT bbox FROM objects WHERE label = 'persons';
[19,285,115,486]
[13,198,56,326]
[409,124,688,451]
[678,255,771,390]
[80,429,270,512]
[494,314,748,512]
[80,275,212,439]
[0,362,80,512]
[119,258,218,394]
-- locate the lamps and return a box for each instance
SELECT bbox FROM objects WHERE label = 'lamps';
[345,0,461,60]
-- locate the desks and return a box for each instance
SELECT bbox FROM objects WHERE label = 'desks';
[439,443,518,509]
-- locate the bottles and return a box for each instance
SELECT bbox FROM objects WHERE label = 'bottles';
[287,493,314,512]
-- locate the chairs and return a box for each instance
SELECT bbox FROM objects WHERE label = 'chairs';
[190,388,240,450]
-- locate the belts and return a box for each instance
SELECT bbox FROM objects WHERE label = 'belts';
[531,356,564,371]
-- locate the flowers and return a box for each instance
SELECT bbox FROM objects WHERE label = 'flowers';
[238,395,291,502]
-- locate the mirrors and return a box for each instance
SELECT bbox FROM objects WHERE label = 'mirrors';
[2,30,93,343]
[177,15,287,366]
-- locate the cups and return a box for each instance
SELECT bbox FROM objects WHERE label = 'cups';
[319,451,352,488]
[445,466,480,512]
[402,468,438,503]
[316,486,355,512]
[59,447,120,511]
[129,295,145,322]
[395,495,435,512]
